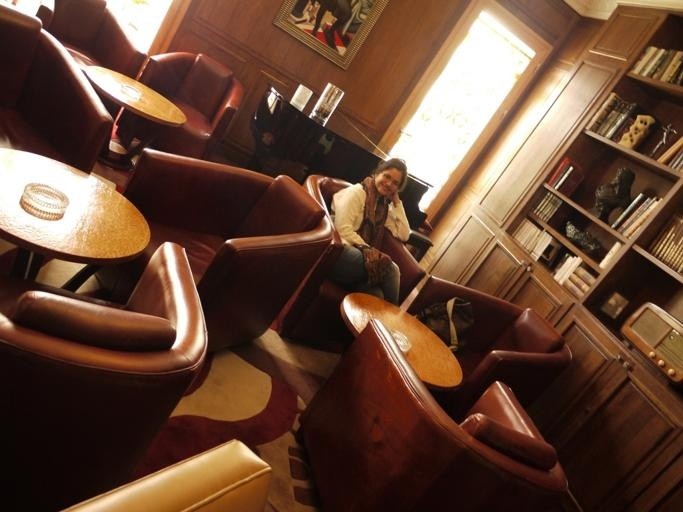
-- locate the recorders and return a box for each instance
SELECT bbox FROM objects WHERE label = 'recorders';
[620,301,683,386]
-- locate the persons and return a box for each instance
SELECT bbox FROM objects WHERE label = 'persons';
[331,158,411,300]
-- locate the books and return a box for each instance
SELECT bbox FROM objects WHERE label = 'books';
[585,46,683,172]
[511,157,683,301]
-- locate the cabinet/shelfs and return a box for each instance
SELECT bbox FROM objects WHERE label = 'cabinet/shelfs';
[425,3,683,510]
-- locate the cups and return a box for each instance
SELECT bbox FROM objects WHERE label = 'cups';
[307,82,344,129]
[289,82,313,114]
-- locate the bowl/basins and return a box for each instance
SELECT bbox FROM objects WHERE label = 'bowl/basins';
[565,219,601,255]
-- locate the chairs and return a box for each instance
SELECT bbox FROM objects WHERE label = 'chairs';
[0,0,578,511]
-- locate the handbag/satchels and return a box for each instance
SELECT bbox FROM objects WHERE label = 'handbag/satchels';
[412,296,474,351]
